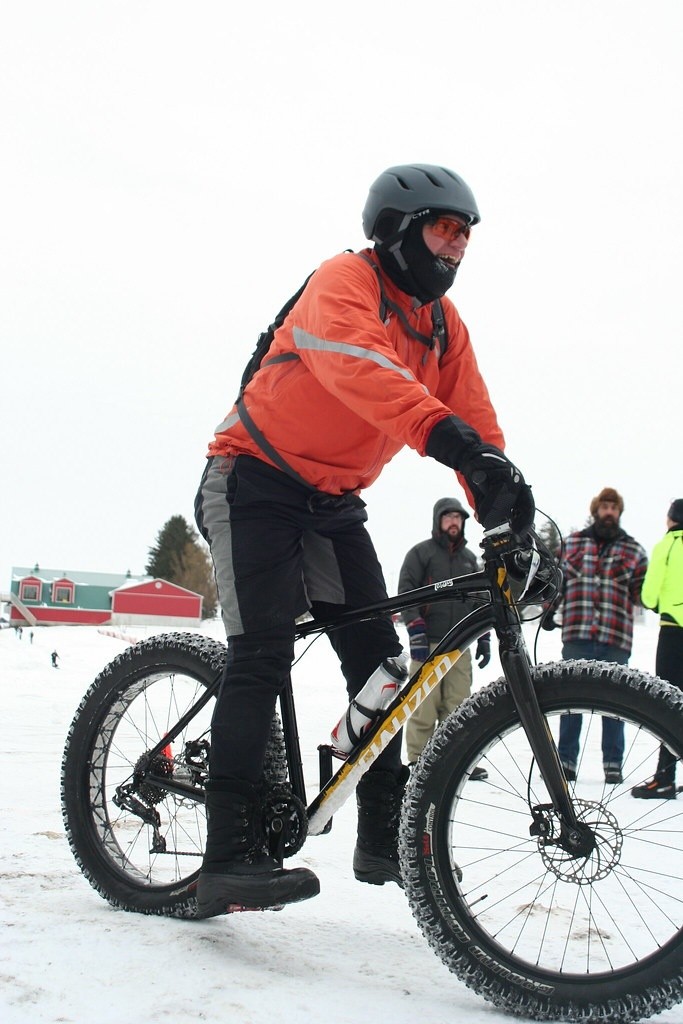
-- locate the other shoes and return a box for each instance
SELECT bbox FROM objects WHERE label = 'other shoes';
[540,767,575,780]
[468,767,489,780]
[606,775,623,784]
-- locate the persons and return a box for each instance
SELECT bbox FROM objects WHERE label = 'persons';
[14,625,60,668]
[539,489,648,782]
[194,167,535,916]
[399,498,490,780]
[631,499,683,799]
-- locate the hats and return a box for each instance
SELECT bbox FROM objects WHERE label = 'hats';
[668,499,683,523]
[589,487,624,517]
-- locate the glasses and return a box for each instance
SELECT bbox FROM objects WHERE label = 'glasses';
[425,218,470,242]
[444,513,465,522]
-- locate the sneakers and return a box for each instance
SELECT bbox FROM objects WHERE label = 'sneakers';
[631,780,676,799]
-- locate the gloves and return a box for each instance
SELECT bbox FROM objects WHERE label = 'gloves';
[425,415,536,532]
[406,624,431,663]
[476,640,491,668]
[541,611,557,630]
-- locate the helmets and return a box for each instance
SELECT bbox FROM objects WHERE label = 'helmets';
[360,164,481,240]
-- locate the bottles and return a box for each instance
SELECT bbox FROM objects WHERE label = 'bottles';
[330,651,409,752]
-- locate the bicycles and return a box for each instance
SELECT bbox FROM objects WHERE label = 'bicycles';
[61,470,683,1024]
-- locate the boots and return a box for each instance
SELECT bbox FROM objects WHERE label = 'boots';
[342,764,462,888]
[192,778,321,919]
